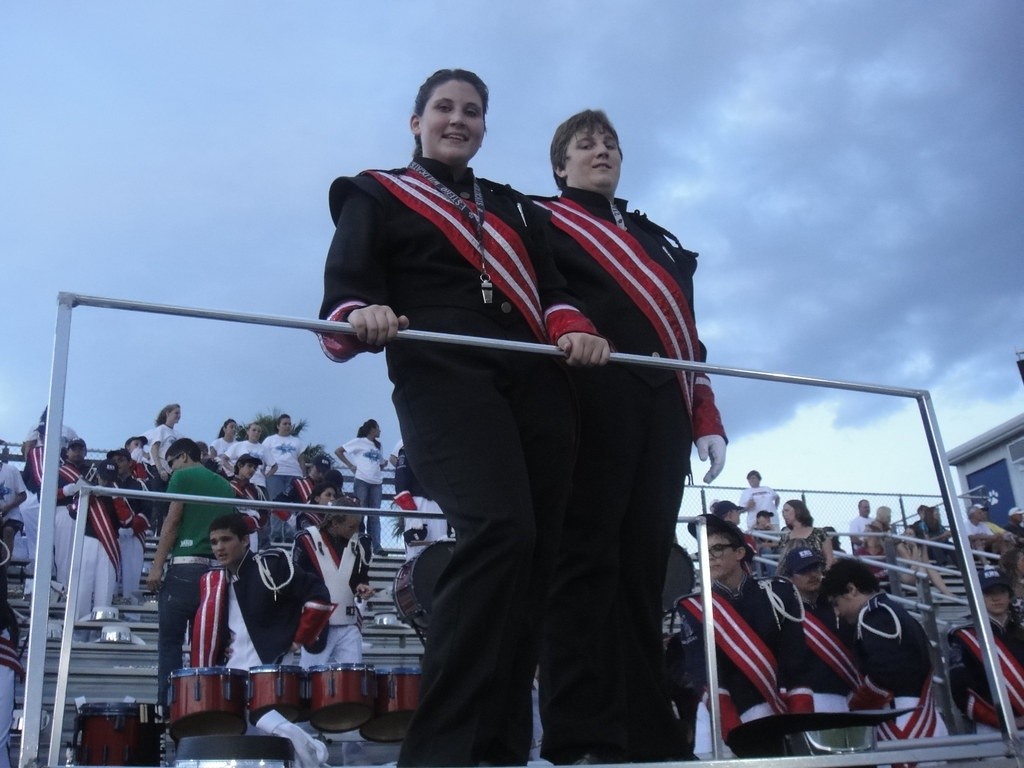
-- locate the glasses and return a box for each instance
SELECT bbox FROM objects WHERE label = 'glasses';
[694,544,740,559]
[791,563,819,573]
[167,451,186,468]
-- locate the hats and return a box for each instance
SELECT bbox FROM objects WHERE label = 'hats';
[977,570,1014,600]
[125,436,148,449]
[97,459,119,482]
[1007,507,1024,516]
[756,511,773,518]
[687,514,747,545]
[713,501,748,518]
[786,548,818,577]
[973,504,984,509]
[107,448,131,461]
[67,438,87,453]
[313,455,331,473]
[235,454,263,466]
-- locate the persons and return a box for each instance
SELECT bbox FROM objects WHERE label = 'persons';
[526,111,728,765]
[0,404,1024,768]
[314,70,614,768]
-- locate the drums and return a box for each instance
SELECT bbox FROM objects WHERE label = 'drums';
[392,537,457,631]
[307,662,379,733]
[167,666,250,741]
[72,701,162,767]
[248,664,303,727]
[359,666,421,743]
[661,542,695,613]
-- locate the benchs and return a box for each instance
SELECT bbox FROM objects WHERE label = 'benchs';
[0,546,1024,768]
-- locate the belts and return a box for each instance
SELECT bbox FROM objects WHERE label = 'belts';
[169,556,221,567]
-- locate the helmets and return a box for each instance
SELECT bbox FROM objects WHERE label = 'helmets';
[980,507,989,512]
